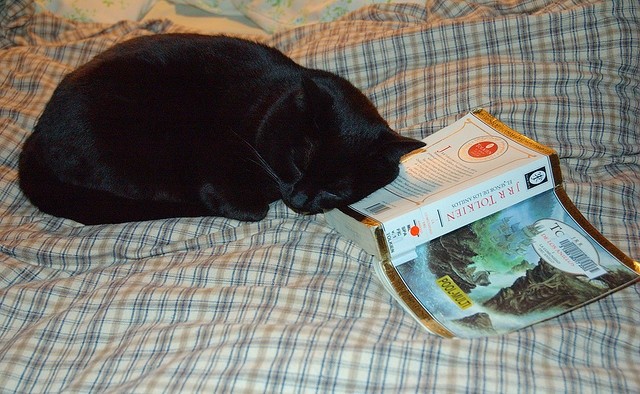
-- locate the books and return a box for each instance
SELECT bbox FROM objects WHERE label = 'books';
[323,105,640,340]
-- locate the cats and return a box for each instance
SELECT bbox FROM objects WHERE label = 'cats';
[17,31,427,226]
